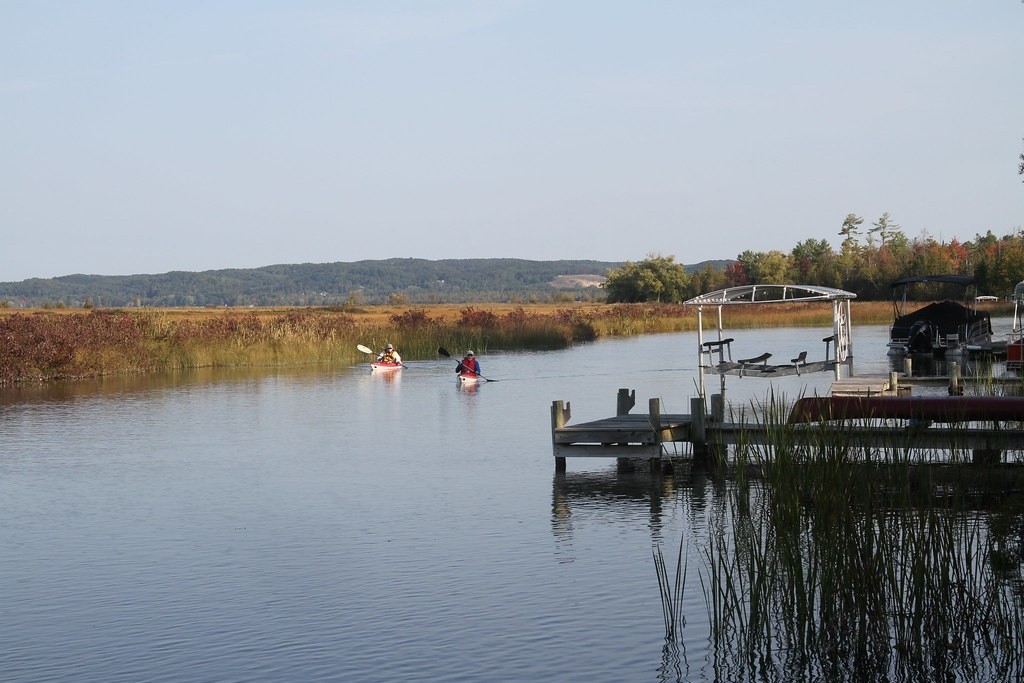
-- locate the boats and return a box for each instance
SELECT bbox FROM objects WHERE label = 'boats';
[456,374,480,387]
[371,361,403,373]
[888,275,993,359]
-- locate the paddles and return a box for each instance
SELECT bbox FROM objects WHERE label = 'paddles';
[438,347,494,382]
[357,344,407,369]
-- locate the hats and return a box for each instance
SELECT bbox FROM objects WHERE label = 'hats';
[387,344,392,348]
[466,350,473,355]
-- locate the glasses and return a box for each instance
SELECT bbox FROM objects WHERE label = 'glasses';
[467,355,473,356]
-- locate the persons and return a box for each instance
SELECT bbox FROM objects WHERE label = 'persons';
[456,350,480,378]
[376,343,400,366]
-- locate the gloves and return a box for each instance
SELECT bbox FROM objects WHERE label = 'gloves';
[458,362,463,367]
[475,371,479,374]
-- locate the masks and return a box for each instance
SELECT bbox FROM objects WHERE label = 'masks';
[387,349,392,352]
